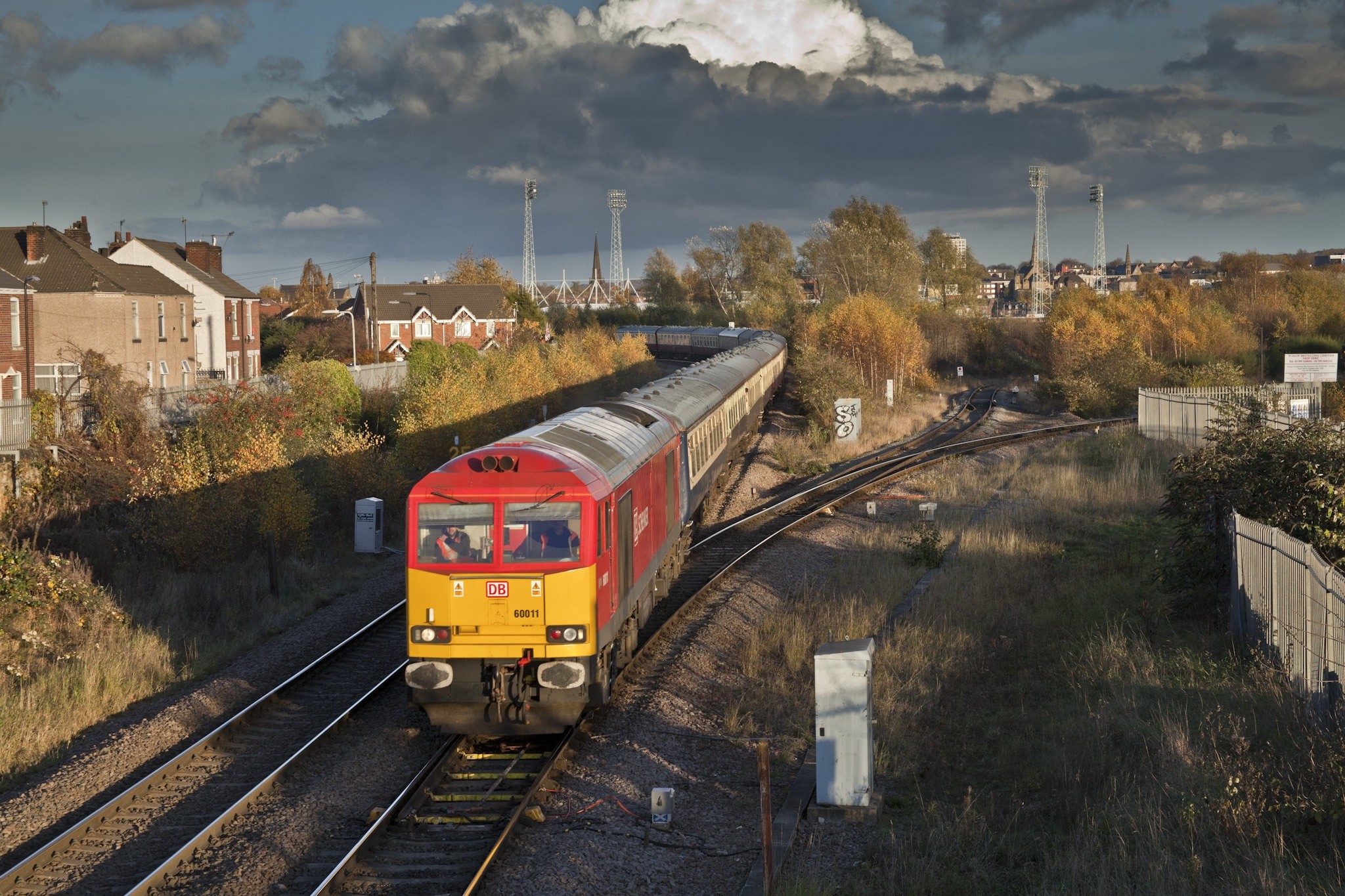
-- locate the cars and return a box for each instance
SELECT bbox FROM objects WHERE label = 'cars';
[1027,308,1044,314]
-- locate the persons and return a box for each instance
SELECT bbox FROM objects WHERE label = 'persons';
[540,520,579,557]
[434,525,472,563]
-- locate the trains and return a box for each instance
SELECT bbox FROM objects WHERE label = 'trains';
[405,325,788,734]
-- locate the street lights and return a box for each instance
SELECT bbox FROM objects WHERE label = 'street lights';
[416,293,433,339]
[322,310,356,366]
[24,277,33,397]
